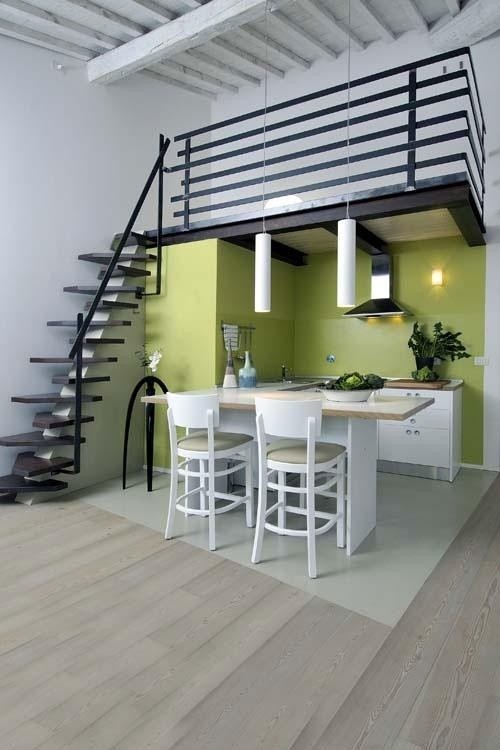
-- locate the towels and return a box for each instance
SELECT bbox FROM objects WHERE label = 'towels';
[222,324,241,351]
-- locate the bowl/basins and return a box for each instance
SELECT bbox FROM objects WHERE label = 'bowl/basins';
[317,388,380,402]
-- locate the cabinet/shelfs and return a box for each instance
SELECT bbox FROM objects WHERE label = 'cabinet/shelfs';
[378,389,461,482]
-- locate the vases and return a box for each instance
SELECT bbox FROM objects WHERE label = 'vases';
[222,338,238,389]
[239,350,256,390]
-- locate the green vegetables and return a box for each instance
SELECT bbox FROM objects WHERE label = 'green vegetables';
[408,321,472,362]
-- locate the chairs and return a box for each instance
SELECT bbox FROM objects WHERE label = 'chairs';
[252,397,348,579]
[164,391,254,551]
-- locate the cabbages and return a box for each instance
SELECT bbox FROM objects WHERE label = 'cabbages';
[340,372,364,389]
[365,374,384,388]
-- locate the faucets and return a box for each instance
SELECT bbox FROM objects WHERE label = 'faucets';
[279,361,288,380]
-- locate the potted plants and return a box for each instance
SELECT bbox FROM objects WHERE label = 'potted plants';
[408,320,471,372]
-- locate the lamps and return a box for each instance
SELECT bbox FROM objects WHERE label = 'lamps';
[255,0,271,312]
[336,0,356,307]
[432,269,443,286]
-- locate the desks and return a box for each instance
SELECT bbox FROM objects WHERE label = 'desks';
[142,387,435,557]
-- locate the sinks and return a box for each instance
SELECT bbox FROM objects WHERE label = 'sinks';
[260,379,282,383]
[294,377,321,383]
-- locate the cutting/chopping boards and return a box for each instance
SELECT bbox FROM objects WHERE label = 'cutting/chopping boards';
[385,378,451,390]
